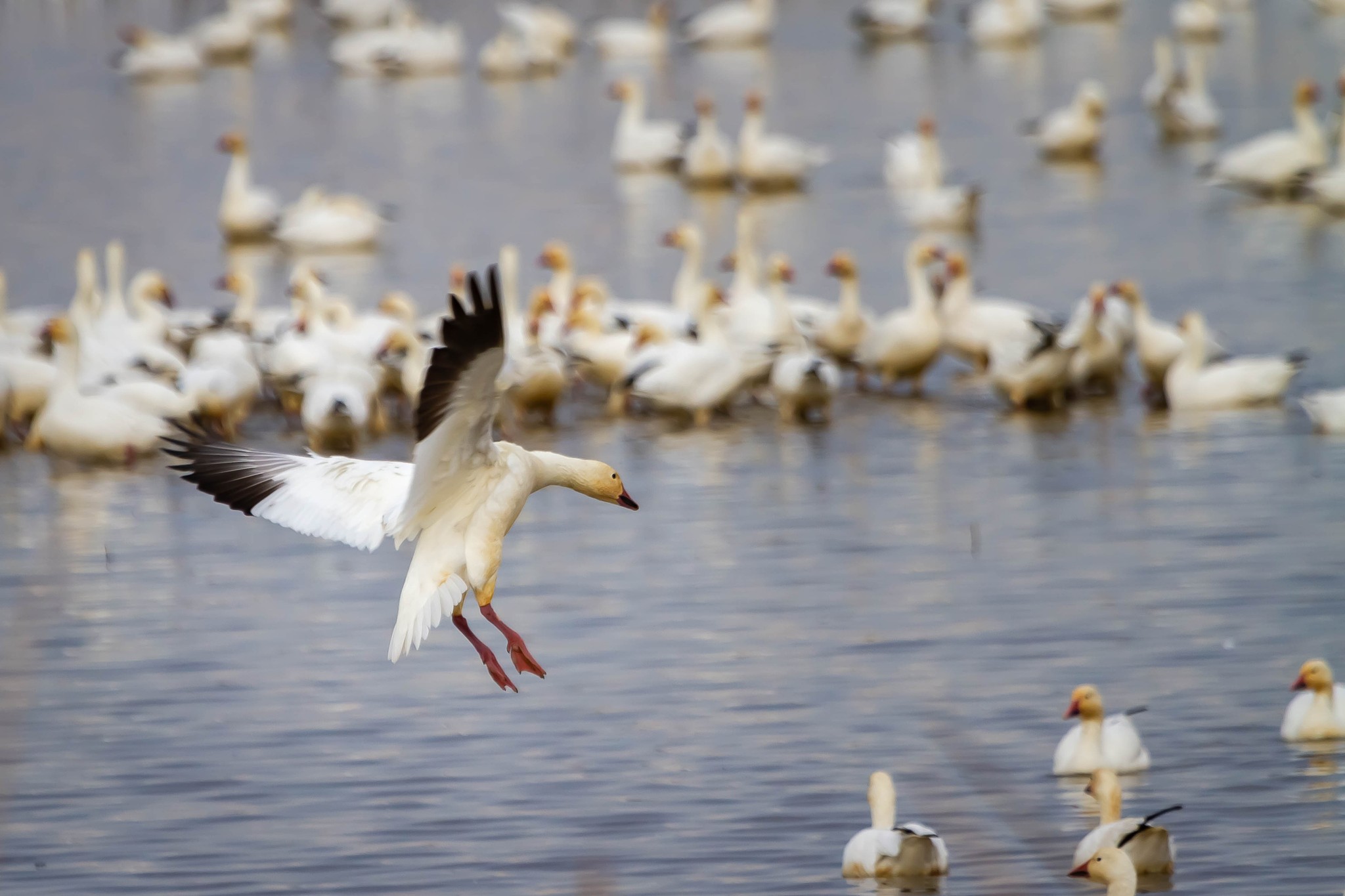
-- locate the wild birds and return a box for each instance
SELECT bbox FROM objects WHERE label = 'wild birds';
[0,0,1345,896]
